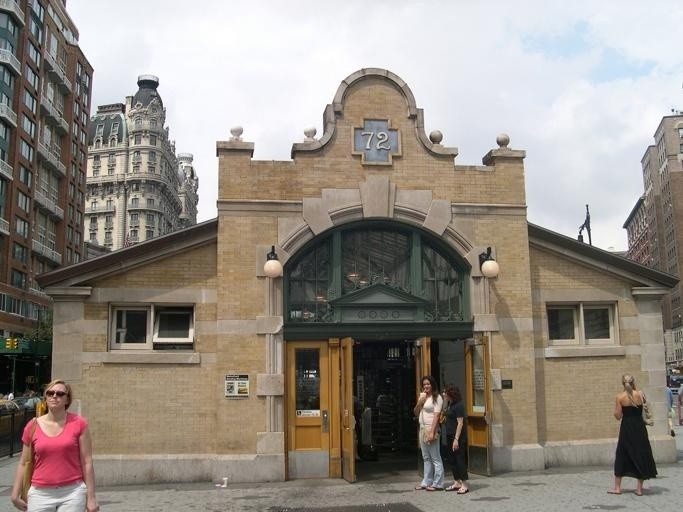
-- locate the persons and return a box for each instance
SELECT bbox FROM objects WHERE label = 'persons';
[441,381,472,494]
[412,375,444,491]
[11,379,102,512]
[666,384,676,438]
[20,386,40,408]
[606,372,659,496]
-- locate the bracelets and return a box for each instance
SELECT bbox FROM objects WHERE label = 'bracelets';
[453,436,458,441]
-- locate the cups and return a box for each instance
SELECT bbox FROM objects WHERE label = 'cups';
[419,393,426,399]
[222,477,228,486]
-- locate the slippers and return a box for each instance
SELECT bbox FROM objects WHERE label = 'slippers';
[414,486,468,494]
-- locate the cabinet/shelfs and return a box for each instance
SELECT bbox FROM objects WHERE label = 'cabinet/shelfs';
[354,361,402,459]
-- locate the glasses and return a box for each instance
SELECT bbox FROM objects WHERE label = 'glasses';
[46,391,67,397]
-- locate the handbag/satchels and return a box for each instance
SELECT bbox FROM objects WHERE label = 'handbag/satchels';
[642,410,653,426]
[20,461,33,504]
[423,428,438,446]
